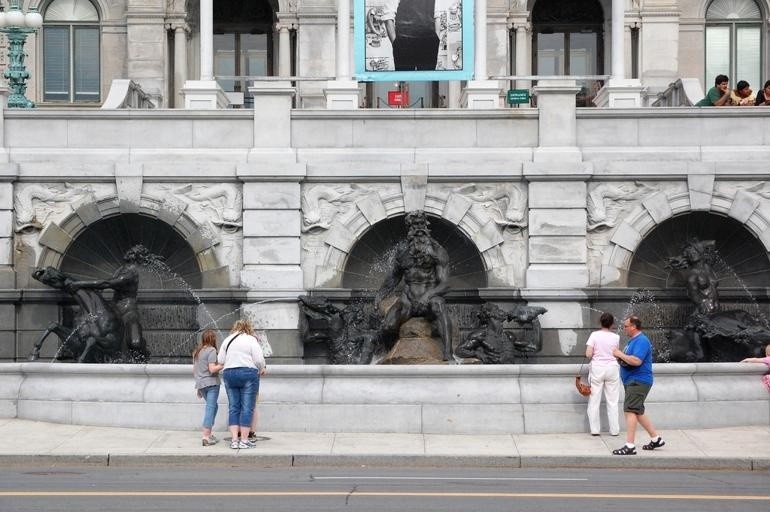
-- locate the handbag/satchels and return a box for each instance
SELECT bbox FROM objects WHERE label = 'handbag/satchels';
[576,376,591,396]
[618,358,632,369]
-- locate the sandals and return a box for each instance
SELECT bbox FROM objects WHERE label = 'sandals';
[613,445,637,455]
[642,437,665,450]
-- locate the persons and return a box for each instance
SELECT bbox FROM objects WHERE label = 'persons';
[738,344,770,394]
[665,242,770,360]
[453,302,544,365]
[612,315,666,455]
[62,244,148,354]
[729,80,756,106]
[380,0,446,71]
[692,74,731,106]
[585,311,623,437]
[235,329,263,441]
[360,209,462,365]
[216,318,266,449]
[755,81,770,106]
[193,330,224,447]
[299,296,377,364]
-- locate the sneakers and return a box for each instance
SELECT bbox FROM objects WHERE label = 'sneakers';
[230,432,256,449]
[203,436,217,446]
